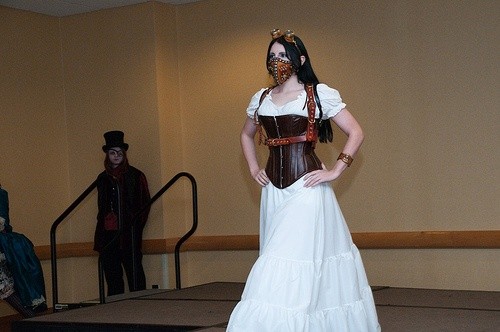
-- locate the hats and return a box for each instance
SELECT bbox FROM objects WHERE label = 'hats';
[101,131,129,153]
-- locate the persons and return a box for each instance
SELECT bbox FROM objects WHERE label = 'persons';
[92,131,151,298]
[223,28,385,332]
[0,184,48,318]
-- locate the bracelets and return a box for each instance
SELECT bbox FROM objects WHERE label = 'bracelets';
[336,152,353,167]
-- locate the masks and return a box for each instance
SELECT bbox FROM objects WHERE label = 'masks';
[268,57,296,85]
